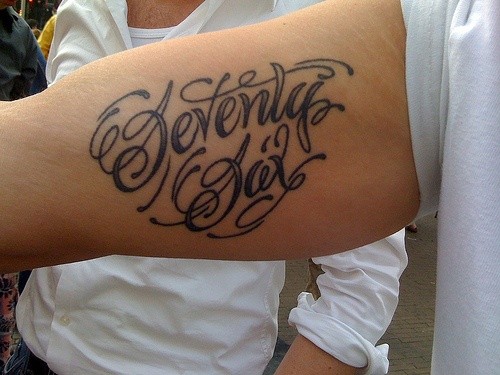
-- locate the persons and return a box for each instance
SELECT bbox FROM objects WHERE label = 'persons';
[0,0,48,374]
[0,0,499,375]
[4,1,410,375]
[8,1,66,96]
